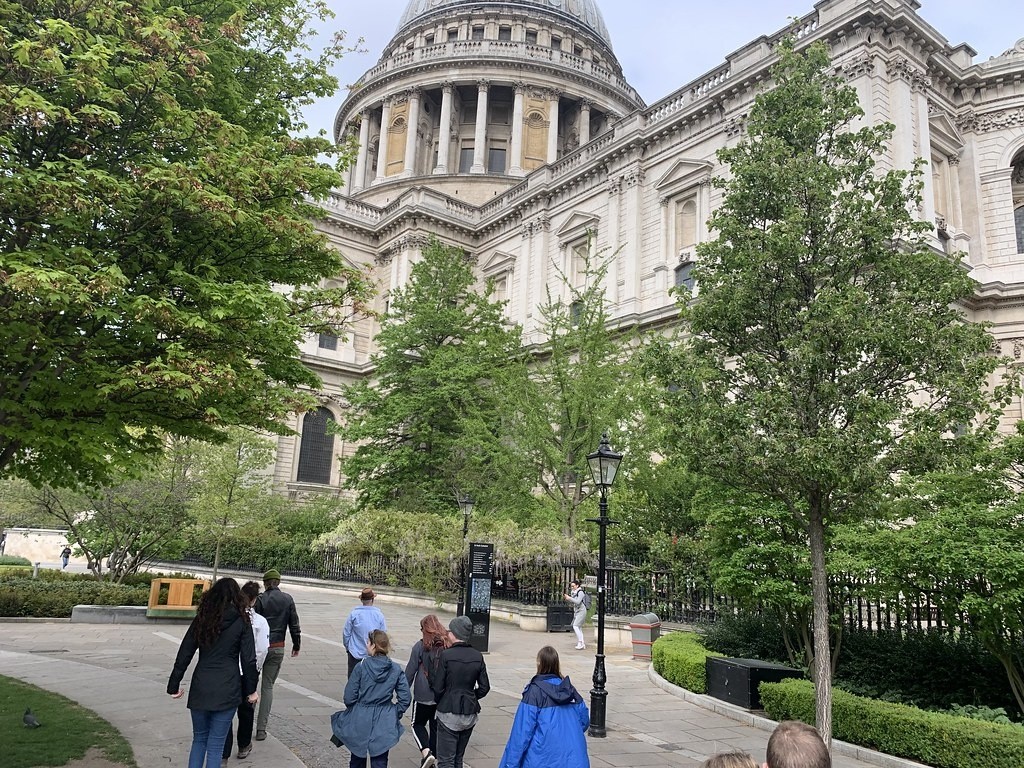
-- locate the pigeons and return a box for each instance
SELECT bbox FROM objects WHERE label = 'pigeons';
[23,708,42,731]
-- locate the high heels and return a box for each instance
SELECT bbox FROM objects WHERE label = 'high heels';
[575,641,586,649]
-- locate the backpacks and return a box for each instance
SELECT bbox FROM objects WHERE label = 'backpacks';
[577,589,592,610]
[420,638,445,690]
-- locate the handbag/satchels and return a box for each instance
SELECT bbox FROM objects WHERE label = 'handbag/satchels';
[330,734,345,748]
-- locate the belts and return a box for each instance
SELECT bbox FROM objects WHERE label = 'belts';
[269,641,285,648]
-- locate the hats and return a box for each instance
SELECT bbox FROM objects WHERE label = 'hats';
[449,615,472,640]
[263,569,281,581]
[359,588,377,600]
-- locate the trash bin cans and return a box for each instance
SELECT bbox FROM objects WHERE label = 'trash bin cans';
[629,613,661,661]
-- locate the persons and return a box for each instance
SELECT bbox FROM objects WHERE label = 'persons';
[59,546,71,569]
[253,569,301,740]
[219,581,271,767]
[498,646,589,768]
[342,588,387,683]
[166,577,259,768]
[329,629,412,768]
[700,721,831,768]
[404,614,452,768]
[563,580,587,649]
[433,615,491,768]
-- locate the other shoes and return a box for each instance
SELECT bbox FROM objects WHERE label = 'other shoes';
[220,758,229,768]
[237,742,252,759]
[256,731,267,741]
[420,750,436,768]
